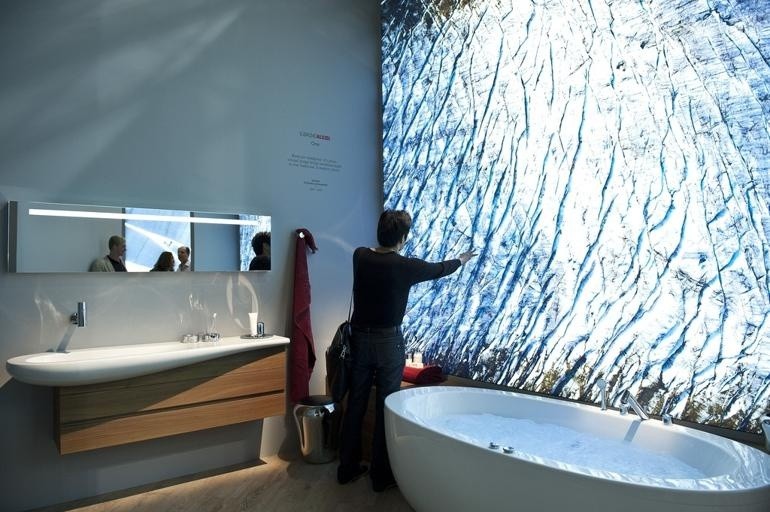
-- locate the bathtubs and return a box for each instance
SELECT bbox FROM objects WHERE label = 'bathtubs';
[383,386,770,512]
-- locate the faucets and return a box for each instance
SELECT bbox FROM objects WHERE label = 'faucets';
[620,389,650,420]
[71,301,87,328]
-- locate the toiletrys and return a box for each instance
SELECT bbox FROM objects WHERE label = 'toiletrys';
[247,312,259,336]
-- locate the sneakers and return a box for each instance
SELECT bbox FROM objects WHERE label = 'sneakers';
[351,463,370,481]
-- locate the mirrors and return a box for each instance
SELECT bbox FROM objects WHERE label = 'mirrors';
[6,200,272,273]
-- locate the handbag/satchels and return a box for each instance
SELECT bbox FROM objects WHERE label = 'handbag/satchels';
[325,321,353,404]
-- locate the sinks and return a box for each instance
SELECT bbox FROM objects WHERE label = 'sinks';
[4,334,291,387]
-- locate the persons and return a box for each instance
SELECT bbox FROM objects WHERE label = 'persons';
[248,231,271,270]
[89,235,130,272]
[335,208,479,493]
[176,246,192,271]
[148,251,175,272]
